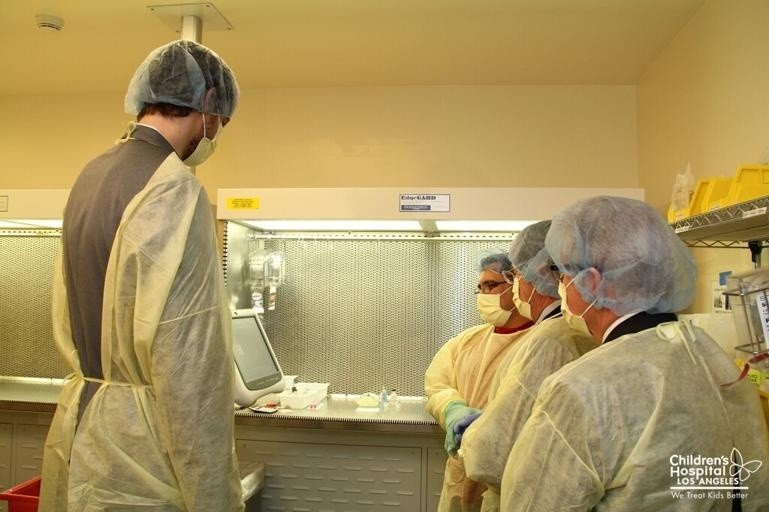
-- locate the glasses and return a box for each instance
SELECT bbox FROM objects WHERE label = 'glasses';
[474,281,507,294]
[501,262,527,286]
[548,261,585,282]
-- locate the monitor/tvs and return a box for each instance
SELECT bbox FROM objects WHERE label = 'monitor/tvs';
[227,307,285,406]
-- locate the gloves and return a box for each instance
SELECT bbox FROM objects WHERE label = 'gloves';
[443,400,484,458]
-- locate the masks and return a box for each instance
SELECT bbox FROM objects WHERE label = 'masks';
[182,120,225,167]
[474,290,512,328]
[511,274,537,324]
[554,280,601,340]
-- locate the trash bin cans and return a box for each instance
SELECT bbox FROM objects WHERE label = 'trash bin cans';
[239,461,265,512]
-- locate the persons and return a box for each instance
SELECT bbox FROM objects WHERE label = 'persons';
[461,219,600,510]
[423,250,534,511]
[499,195,769,512]
[38,39,246,511]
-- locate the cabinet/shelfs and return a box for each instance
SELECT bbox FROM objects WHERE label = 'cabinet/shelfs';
[0,400,446,512]
